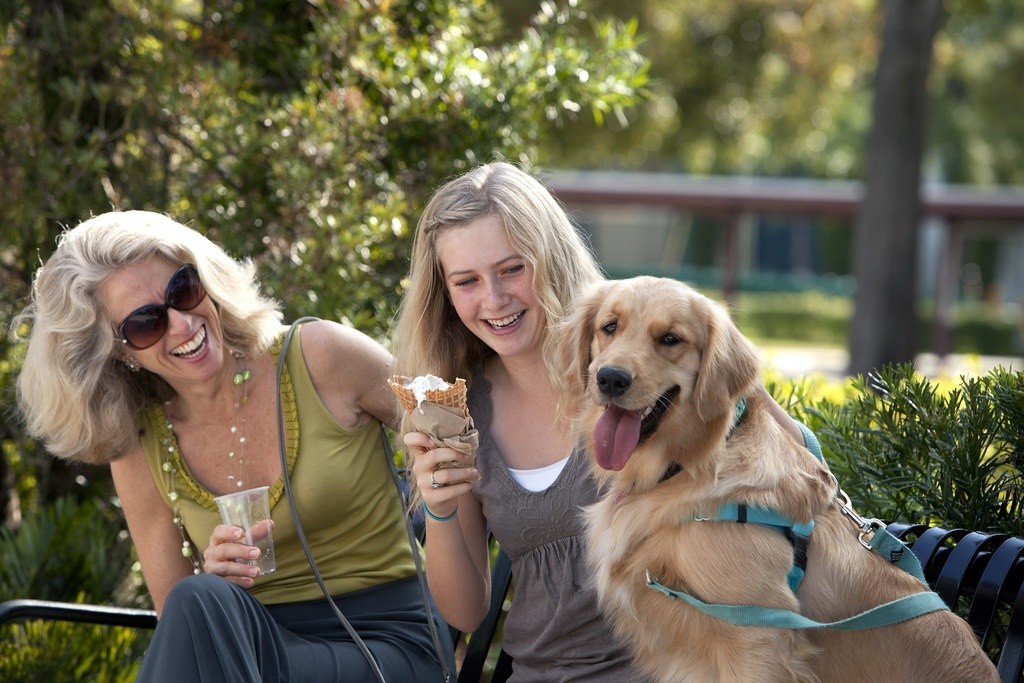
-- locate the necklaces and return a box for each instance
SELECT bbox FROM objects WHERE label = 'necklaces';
[162,357,251,577]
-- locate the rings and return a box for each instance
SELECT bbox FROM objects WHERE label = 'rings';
[431,469,444,489]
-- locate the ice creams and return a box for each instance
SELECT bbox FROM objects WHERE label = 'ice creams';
[387,374,468,416]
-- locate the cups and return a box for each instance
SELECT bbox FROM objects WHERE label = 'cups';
[213,486,277,576]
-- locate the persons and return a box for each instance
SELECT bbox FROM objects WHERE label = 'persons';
[391,161,823,683]
[15,210,465,683]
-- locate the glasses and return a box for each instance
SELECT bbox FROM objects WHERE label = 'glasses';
[110,261,209,350]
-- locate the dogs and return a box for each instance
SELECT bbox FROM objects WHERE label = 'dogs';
[549,274,998,683]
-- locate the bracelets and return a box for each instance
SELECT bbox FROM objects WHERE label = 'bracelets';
[421,500,458,522]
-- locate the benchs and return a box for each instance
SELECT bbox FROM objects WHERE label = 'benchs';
[0,466,1024,683]
